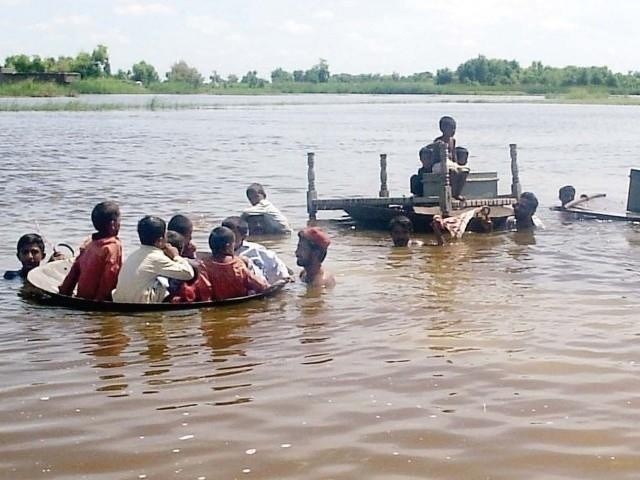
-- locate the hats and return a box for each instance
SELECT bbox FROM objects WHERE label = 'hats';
[298,227,331,248]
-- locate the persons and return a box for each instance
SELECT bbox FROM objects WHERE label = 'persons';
[3,199,291,305]
[387,214,446,247]
[558,185,576,207]
[409,147,433,197]
[505,191,538,230]
[240,181,293,236]
[293,226,335,290]
[432,116,471,200]
[455,147,469,165]
